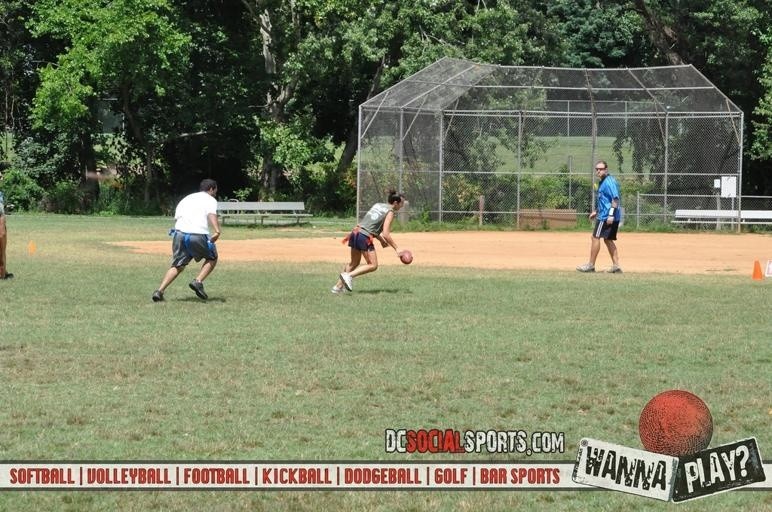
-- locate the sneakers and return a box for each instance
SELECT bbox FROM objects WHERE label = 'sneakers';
[189,279,209,300]
[576,262,596,272]
[339,272,353,292]
[331,284,347,295]
[151,290,165,301]
[602,264,622,274]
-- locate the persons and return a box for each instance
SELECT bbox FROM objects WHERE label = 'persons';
[574,161,623,273]
[153,179,220,301]
[0,193,15,279]
[331,189,405,295]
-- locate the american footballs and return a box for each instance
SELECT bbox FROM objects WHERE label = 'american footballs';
[399,250,414,265]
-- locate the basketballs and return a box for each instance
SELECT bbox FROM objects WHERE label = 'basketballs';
[639,390,713,456]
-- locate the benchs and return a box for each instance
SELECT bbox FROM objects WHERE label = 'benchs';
[671,209,771,233]
[216,201,314,225]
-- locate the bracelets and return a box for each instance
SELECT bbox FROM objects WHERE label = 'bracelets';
[607,207,616,216]
[214,231,220,234]
